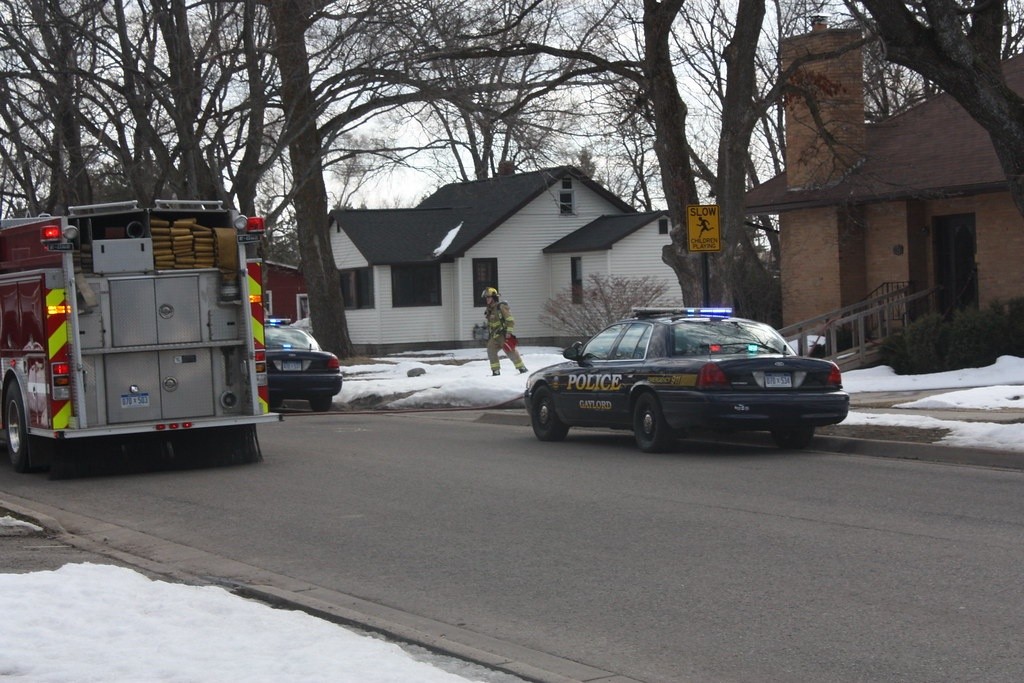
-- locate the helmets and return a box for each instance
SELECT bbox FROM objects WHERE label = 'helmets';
[481,286,502,297]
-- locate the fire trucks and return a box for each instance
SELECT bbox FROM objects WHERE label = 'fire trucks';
[0,200,284,471]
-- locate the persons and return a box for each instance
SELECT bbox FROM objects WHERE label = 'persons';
[482,288,527,376]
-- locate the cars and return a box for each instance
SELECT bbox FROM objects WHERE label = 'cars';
[523,314,849,452]
[264,324,342,411]
